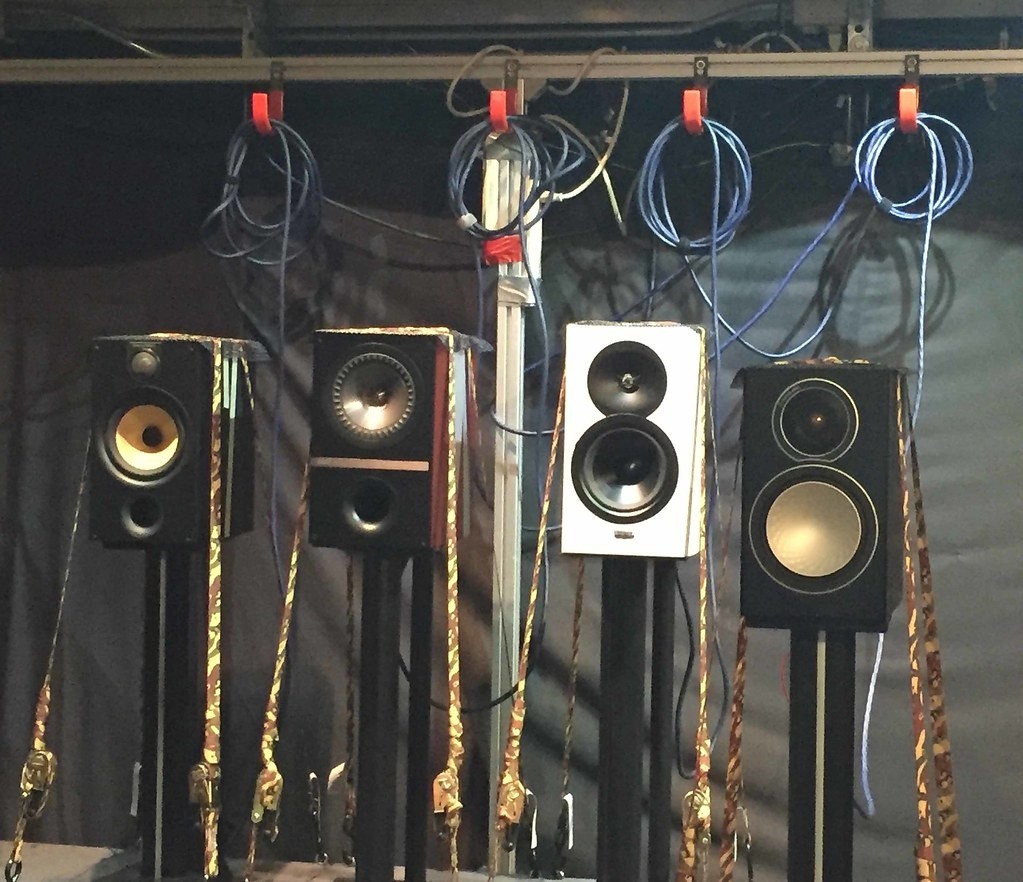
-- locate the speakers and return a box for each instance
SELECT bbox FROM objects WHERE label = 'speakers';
[86,318,914,634]
